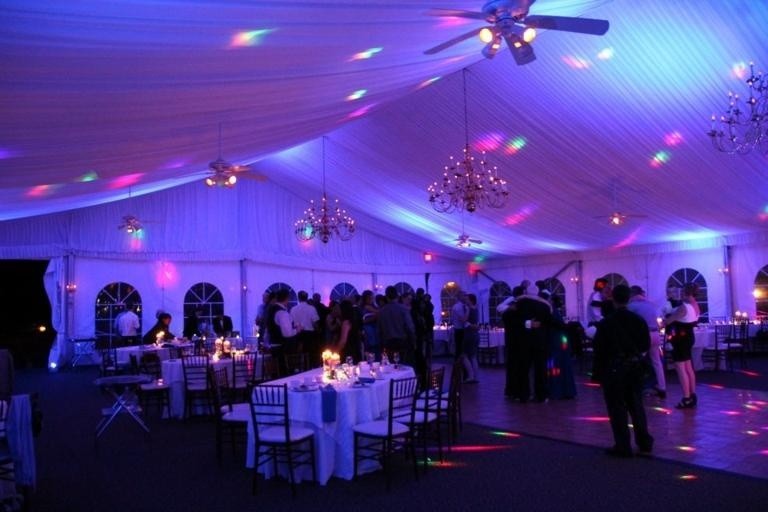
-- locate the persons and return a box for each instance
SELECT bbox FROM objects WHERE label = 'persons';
[494,279,578,403]
[592,285,655,456]
[213,309,233,337]
[184,307,203,331]
[659,281,701,409]
[119,301,140,344]
[587,282,604,321]
[256,285,479,384]
[628,285,666,399]
[661,283,681,352]
[143,312,174,344]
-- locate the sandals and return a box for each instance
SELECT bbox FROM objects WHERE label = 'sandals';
[673,393,697,409]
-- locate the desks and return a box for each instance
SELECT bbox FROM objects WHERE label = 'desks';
[90,375,151,436]
[68,335,100,367]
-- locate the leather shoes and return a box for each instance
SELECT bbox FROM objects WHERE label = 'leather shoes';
[500,391,576,404]
[648,384,666,399]
[461,375,480,385]
[611,435,655,461]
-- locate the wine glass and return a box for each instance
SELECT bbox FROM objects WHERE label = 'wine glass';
[290,349,399,391]
[141,330,200,349]
[696,311,752,331]
[197,338,251,360]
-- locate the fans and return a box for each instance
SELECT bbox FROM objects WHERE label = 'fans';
[99,184,164,227]
[171,119,270,188]
[442,204,482,244]
[411,0,608,66]
[590,182,649,221]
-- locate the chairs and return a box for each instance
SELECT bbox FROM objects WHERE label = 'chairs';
[29,392,46,469]
[480,326,497,366]
[657,331,670,367]
[353,360,462,486]
[0,349,18,402]
[171,339,319,489]
[130,351,172,426]
[713,316,767,372]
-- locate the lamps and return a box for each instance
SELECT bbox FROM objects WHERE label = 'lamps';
[427,66,511,213]
[124,224,142,234]
[455,239,470,249]
[477,14,538,51]
[705,43,768,158]
[201,172,237,188]
[608,211,627,225]
[293,135,357,243]
